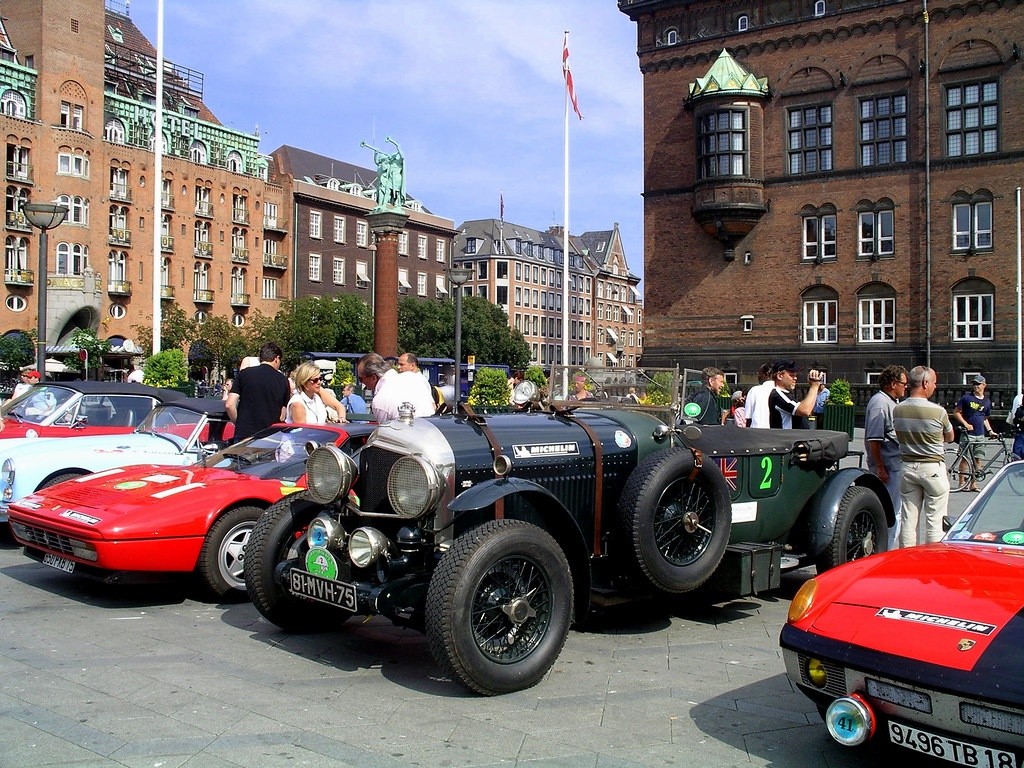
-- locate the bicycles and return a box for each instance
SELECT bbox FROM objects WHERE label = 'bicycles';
[939,426,1023,495]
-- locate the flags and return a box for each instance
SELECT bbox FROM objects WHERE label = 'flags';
[563,34,584,119]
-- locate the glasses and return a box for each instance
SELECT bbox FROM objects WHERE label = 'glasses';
[21,375,28,377]
[895,381,908,386]
[308,377,322,383]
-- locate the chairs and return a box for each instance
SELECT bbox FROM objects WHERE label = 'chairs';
[111,408,136,428]
[87,404,108,425]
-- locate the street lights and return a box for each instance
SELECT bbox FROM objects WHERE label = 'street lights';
[20,198,69,382]
[441,266,476,414]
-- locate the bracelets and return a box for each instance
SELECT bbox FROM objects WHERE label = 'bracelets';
[987,429,993,433]
[0,418,4,421]
[339,416,345,419]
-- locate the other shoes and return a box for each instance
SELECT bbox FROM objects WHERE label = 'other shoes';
[960,488,970,492]
[971,488,981,492]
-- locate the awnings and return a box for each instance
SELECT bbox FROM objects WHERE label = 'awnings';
[302,351,456,363]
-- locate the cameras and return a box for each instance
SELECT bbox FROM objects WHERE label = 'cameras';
[806,373,826,384]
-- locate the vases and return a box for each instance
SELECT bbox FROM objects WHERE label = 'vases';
[333,384,344,401]
[169,381,197,397]
[642,407,670,426]
[822,403,854,443]
[720,395,732,409]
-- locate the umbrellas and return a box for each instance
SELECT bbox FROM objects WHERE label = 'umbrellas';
[19,357,81,382]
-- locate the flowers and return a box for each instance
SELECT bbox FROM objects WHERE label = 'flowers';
[826,379,854,406]
[719,380,731,397]
[144,345,186,389]
[639,371,671,406]
[465,368,512,405]
[330,358,355,385]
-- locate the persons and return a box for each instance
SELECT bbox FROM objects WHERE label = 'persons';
[373,146,404,205]
[506,369,524,389]
[11,369,48,422]
[893,365,955,548]
[567,376,595,410]
[86,404,109,425]
[1011,387,1024,462]
[813,383,831,429]
[221,342,436,469]
[954,374,1001,491]
[0,398,6,432]
[768,358,824,429]
[627,387,653,405]
[683,364,776,428]
[864,364,910,552]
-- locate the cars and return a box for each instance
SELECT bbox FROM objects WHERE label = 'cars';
[6,420,385,605]
[0,376,235,450]
[0,396,264,529]
[780,459,1024,768]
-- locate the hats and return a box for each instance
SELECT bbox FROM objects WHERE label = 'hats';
[772,359,801,372]
[128,370,144,383]
[27,371,41,378]
[971,376,985,383]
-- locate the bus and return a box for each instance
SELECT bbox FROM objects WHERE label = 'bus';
[295,351,510,407]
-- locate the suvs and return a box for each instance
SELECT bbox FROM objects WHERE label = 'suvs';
[243,359,901,697]
